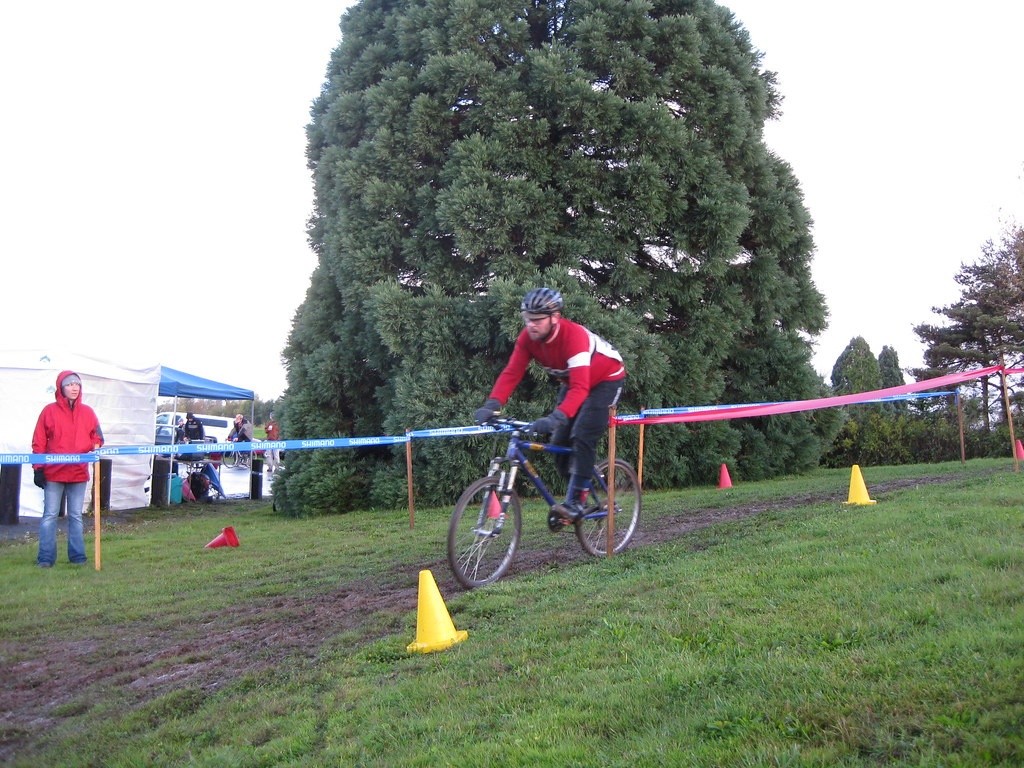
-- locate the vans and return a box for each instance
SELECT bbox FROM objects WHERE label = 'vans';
[155,412,235,456]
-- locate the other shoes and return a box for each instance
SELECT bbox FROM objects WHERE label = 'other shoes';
[242,461,248,464]
[39,562,53,568]
[267,469,272,471]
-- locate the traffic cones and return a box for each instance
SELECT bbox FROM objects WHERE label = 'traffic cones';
[407,570,468,654]
[717,463,734,490]
[204,526,240,548]
[841,465,876,505]
[1015,440,1024,460]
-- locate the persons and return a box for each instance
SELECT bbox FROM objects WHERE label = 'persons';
[183,412,205,441]
[174,418,185,444]
[265,411,280,473]
[474,288,626,519]
[32,371,105,569]
[228,414,253,465]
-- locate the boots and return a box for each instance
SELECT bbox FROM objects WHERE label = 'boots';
[551,474,592,519]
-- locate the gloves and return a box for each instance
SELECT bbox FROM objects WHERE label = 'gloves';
[532,409,569,434]
[474,399,499,424]
[34,468,47,489]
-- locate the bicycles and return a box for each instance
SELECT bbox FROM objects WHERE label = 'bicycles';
[223,438,251,468]
[446,412,643,589]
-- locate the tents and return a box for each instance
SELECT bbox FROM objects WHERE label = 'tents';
[0,343,255,517]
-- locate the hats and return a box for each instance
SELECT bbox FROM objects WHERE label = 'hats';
[61,374,81,387]
[522,311,551,319]
[186,413,194,419]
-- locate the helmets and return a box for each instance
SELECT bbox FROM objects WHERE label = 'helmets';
[521,288,564,313]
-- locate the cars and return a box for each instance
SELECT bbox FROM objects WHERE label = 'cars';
[253,439,268,458]
[156,424,217,455]
